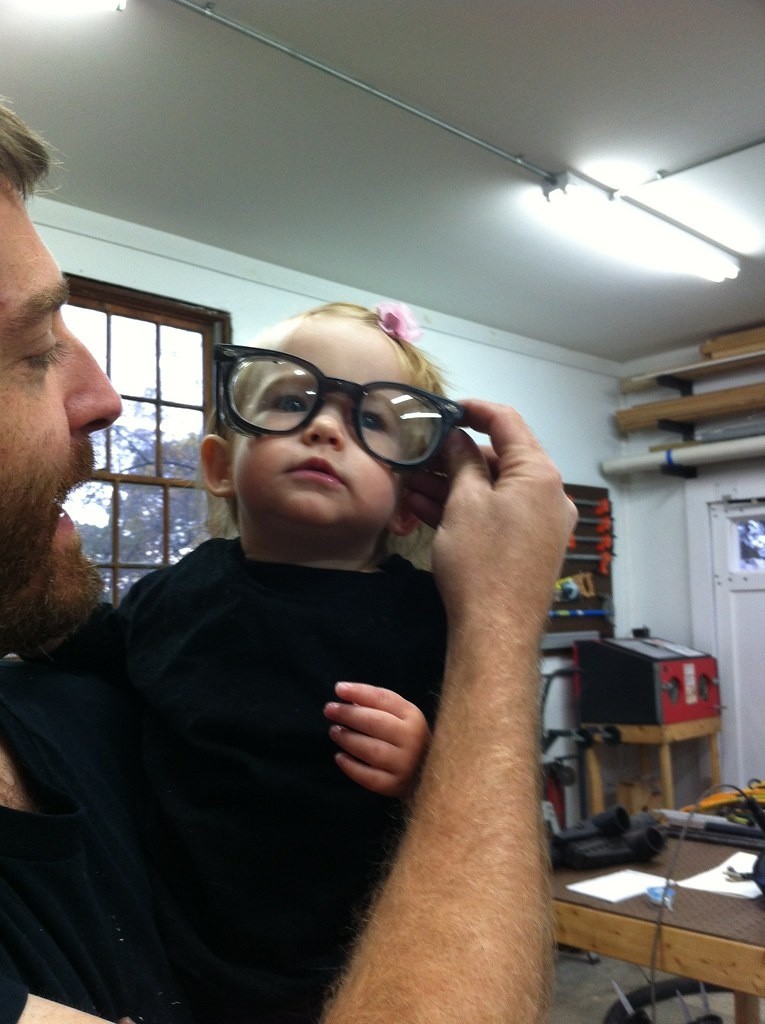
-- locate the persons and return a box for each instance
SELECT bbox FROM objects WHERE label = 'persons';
[55,303,448,1024]
[0,101,577,1024]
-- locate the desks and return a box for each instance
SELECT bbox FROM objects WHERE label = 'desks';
[580,715,722,823]
[550,806,765,1024]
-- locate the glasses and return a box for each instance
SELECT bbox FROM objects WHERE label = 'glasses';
[212,342,465,469]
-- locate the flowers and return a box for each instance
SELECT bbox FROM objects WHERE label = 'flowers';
[376,303,423,341]
